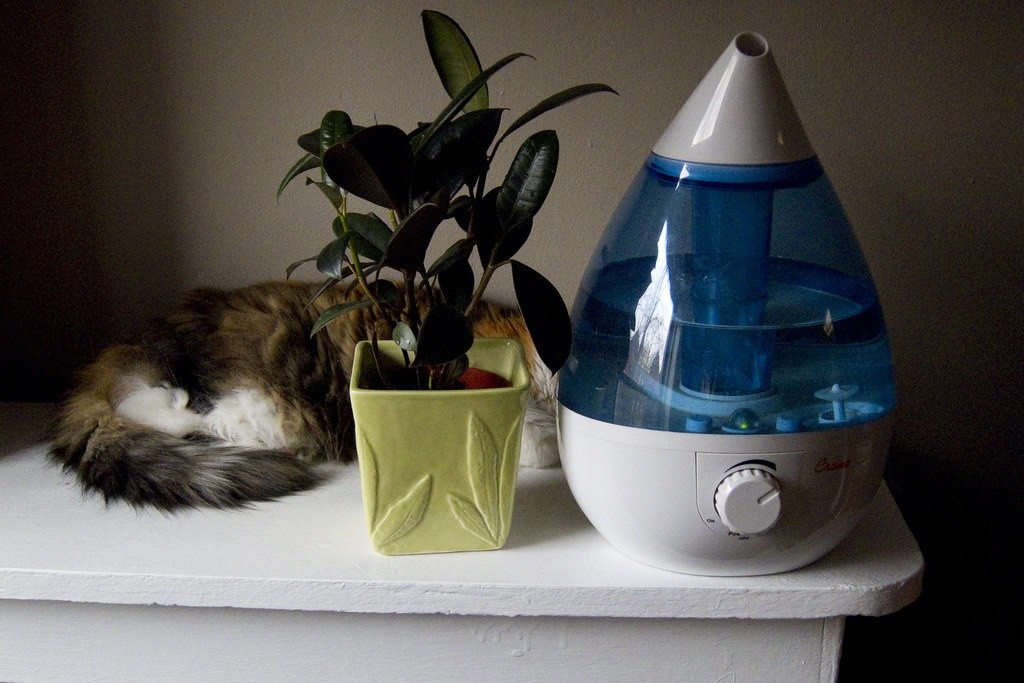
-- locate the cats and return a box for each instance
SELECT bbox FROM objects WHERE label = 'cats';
[42,281,560,516]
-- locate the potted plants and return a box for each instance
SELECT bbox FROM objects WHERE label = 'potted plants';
[274,9,621,556]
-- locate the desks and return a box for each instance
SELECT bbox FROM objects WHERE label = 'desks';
[0,401,924,683]
